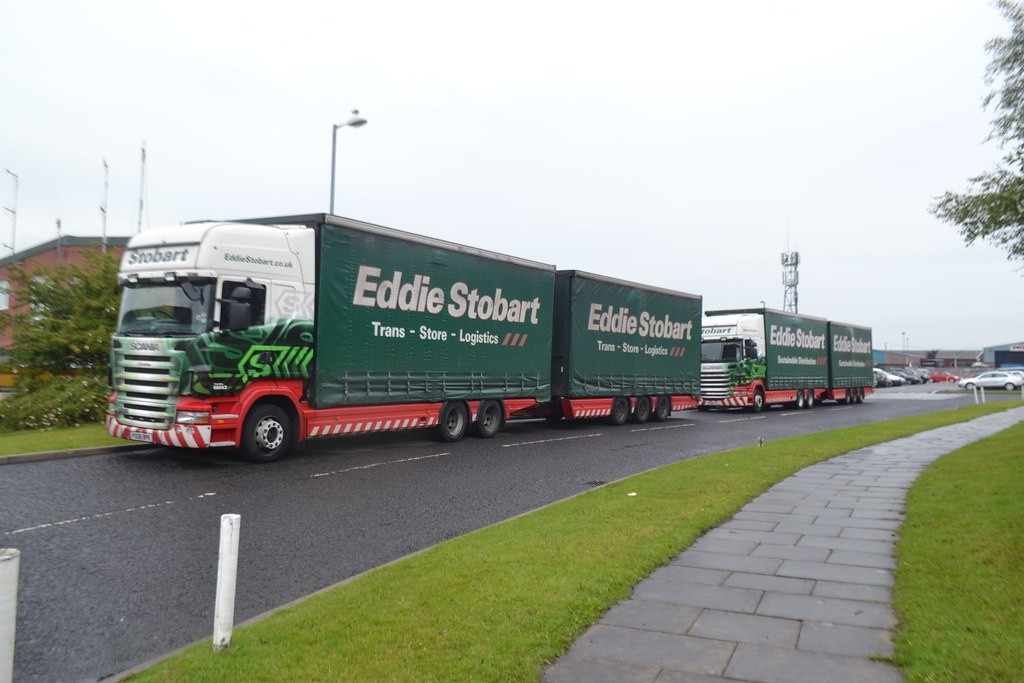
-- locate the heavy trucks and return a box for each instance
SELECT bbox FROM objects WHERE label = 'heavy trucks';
[106,214,703,463]
[701,309,873,412]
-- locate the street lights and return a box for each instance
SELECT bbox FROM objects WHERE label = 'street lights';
[760,300,766,308]
[54,217,63,269]
[328,114,368,217]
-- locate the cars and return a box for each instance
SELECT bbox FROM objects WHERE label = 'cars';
[957,367,1024,390]
[873,367,960,388]
